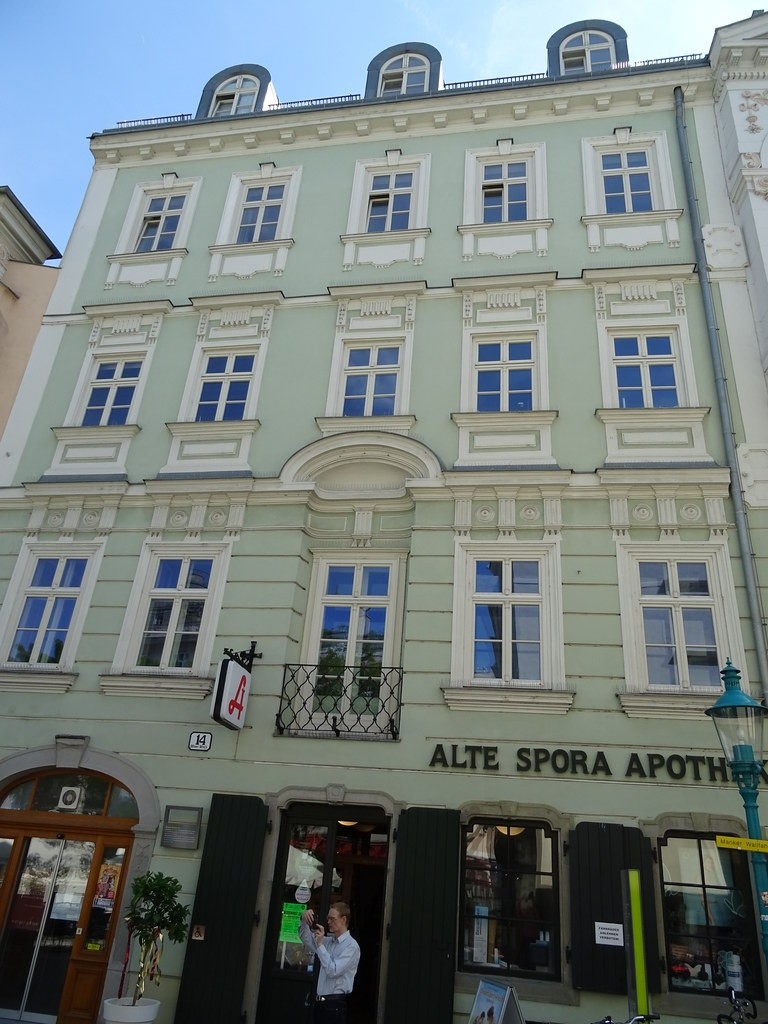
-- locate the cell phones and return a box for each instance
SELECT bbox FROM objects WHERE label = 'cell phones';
[312,914,319,930]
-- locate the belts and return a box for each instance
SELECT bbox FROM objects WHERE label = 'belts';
[316,995,345,1000]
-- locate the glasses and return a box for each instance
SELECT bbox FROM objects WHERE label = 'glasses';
[326,916,337,920]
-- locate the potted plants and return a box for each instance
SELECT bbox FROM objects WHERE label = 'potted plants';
[102,868,191,1024]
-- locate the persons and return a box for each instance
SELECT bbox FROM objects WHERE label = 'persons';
[300,901,361,1024]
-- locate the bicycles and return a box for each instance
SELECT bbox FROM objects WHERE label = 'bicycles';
[716,987,757,1024]
[589,1014,661,1024]
